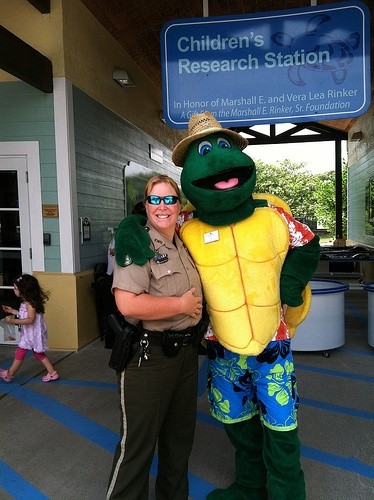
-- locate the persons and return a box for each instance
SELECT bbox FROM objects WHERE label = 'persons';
[105,175,207,500]
[0,274,59,383]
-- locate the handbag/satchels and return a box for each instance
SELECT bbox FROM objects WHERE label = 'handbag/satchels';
[0,315,17,343]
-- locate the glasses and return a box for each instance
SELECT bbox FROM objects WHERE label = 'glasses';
[145,195,181,205]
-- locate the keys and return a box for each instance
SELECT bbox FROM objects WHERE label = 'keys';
[135,339,149,368]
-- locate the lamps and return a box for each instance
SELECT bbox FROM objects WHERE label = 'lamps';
[350,131,363,142]
[112,67,137,88]
[159,110,166,124]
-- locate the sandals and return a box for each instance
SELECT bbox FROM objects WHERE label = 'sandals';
[42,371,59,382]
[0,368,14,382]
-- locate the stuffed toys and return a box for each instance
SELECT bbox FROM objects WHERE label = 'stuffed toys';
[116,113,320,500]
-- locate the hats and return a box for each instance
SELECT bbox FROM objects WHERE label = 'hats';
[172,111,249,167]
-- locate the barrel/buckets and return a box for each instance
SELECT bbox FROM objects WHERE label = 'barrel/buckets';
[362,284,374,347]
[288,278,349,350]
